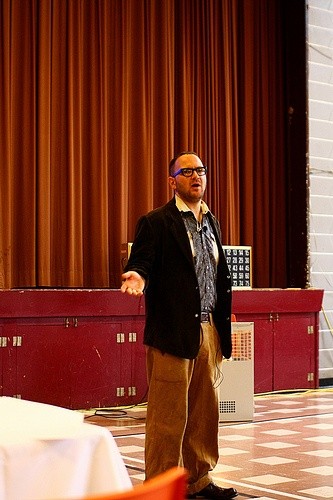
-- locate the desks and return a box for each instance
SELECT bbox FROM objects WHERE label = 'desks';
[0,409,133,500]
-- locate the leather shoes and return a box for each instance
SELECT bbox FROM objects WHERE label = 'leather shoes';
[188,481,239,500]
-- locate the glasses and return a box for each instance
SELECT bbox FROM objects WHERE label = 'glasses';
[172,166,207,177]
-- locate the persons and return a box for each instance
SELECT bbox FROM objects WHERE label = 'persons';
[121,151,238,500]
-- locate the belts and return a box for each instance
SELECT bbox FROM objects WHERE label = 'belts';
[201,313,210,322]
